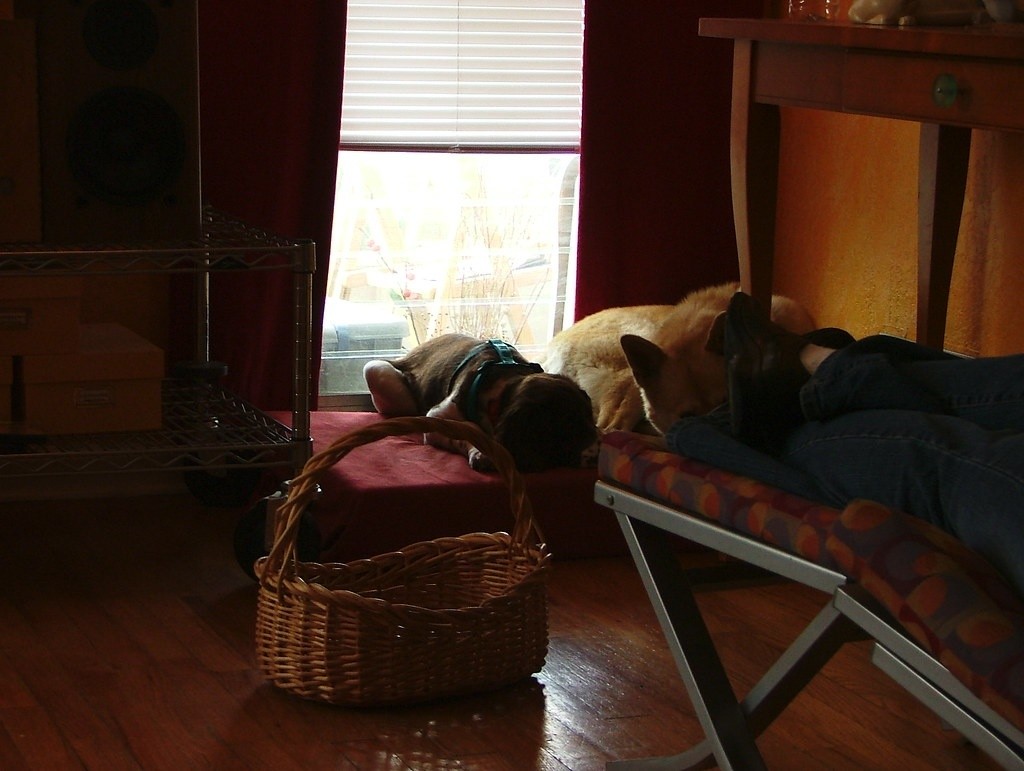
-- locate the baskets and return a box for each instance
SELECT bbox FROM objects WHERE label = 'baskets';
[255,417,554,708]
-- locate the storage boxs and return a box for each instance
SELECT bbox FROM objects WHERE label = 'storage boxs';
[0,269,167,435]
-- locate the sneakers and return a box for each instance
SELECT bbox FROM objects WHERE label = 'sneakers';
[725,291,813,442]
[804,327,855,350]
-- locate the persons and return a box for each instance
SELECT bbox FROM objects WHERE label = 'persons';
[667,292,1024,601]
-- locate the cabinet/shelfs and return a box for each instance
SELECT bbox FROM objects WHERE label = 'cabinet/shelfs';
[0,205,322,583]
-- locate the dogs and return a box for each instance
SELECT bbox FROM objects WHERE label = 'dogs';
[362,334,605,472]
[532,280,820,471]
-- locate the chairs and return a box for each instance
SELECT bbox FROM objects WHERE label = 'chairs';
[597,428,1024,771]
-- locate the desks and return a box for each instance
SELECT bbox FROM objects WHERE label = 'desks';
[697,18,1024,354]
